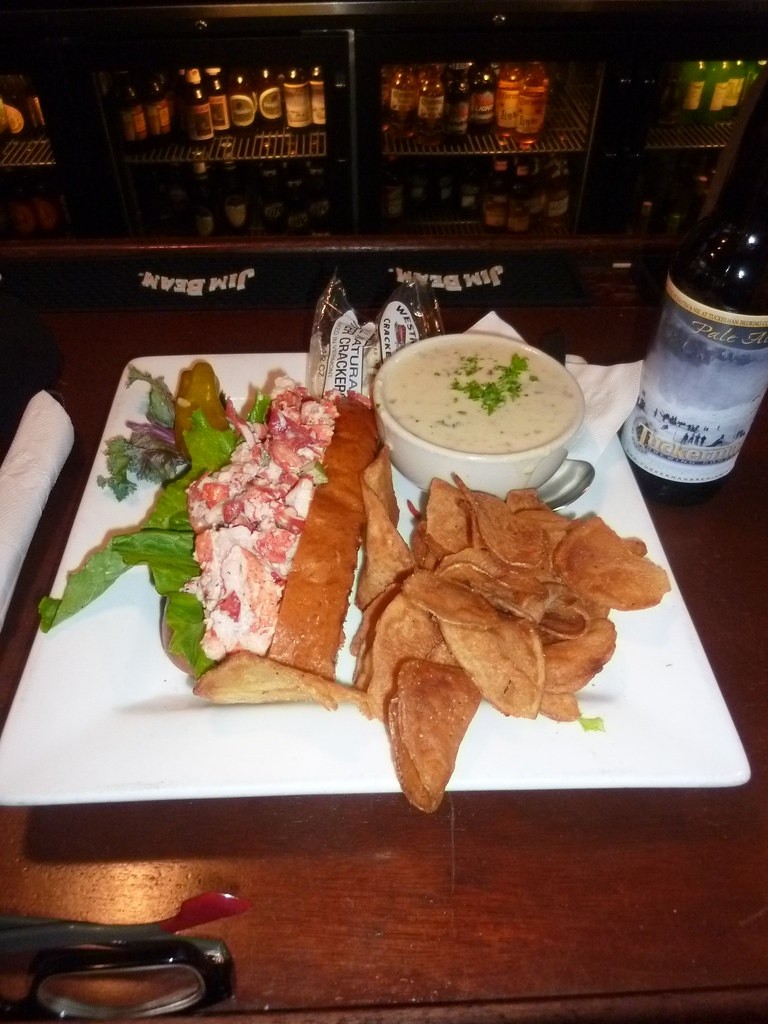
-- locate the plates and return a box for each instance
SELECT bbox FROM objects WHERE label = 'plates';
[0,355,752,807]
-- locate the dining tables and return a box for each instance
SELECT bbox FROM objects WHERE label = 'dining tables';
[0,305,768,1024]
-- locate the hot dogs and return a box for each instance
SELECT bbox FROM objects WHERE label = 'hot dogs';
[39,366,385,680]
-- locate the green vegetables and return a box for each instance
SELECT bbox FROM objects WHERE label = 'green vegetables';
[435,352,538,416]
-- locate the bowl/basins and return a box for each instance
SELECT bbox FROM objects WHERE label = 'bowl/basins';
[372,333,586,502]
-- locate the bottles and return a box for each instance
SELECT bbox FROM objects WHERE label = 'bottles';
[0,76,67,239]
[114,67,329,237]
[382,60,570,234]
[632,60,768,505]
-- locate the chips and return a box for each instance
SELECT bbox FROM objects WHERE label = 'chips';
[192,445,674,813]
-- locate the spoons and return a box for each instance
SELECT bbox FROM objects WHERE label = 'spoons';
[538,459,595,512]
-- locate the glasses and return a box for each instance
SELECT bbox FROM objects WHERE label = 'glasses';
[0,891,253,1023]
